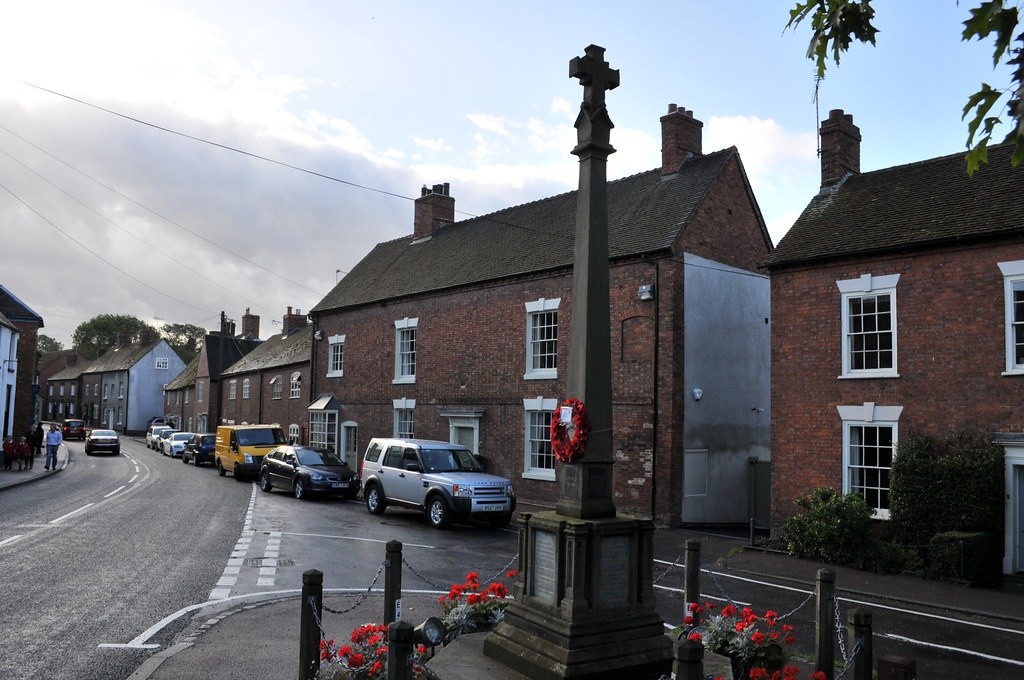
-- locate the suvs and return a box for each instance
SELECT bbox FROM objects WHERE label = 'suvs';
[361,438,516,528]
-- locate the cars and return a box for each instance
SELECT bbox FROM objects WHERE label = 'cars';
[61,419,86,441]
[259,445,361,500]
[85,429,120,456]
[146,425,172,449]
[155,429,181,453]
[163,432,196,458]
[146,417,164,431]
[182,433,216,466]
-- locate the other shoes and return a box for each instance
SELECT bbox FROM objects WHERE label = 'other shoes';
[3,468,7,470]
[18,469,21,471]
[24,467,28,470]
[29,467,33,469]
[8,468,11,471]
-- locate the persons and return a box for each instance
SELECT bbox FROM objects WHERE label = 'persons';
[24,426,37,469]
[45,423,60,455]
[44,424,63,470]
[166,419,174,429]
[36,421,44,454]
[3,435,17,470]
[16,436,31,470]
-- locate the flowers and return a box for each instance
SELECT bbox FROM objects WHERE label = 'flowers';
[549,398,587,463]
[316,571,835,679]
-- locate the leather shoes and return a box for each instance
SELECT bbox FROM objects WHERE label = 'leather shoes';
[53,467,55,470]
[44,466,49,470]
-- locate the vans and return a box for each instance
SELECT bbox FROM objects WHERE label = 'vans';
[214,425,289,482]
